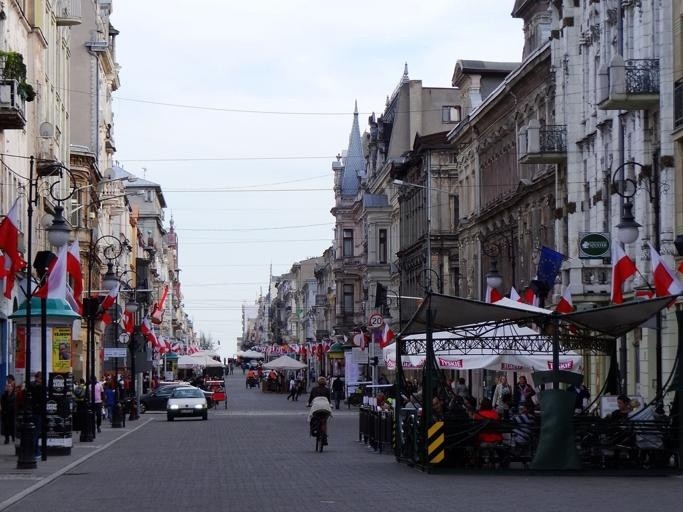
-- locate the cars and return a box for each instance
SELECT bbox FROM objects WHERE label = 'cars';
[125,383,214,413]
[166,386,208,422]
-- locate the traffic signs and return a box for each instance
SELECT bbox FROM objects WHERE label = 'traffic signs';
[370,315,383,328]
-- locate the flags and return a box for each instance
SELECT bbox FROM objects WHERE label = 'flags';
[260,322,394,361]
[486,240,683,334]
[0,195,199,356]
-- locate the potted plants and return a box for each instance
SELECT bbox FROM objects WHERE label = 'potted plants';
[0,50,36,106]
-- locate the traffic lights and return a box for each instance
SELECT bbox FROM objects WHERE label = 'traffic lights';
[369,357,379,366]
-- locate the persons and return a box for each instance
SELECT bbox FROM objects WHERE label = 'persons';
[355,373,540,470]
[566,374,590,450]
[332,375,345,409]
[308,376,331,444]
[229,359,305,401]
[605,397,631,421]
[0,372,159,444]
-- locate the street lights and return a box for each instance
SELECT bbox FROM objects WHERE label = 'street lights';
[77,215,128,445]
[110,270,140,429]
[390,179,460,297]
[608,147,668,424]
[15,155,76,470]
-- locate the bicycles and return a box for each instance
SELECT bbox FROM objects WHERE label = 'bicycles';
[305,403,336,453]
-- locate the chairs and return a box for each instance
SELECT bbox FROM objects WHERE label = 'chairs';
[356,378,683,480]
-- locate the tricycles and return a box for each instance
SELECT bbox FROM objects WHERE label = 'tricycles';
[206,380,228,409]
